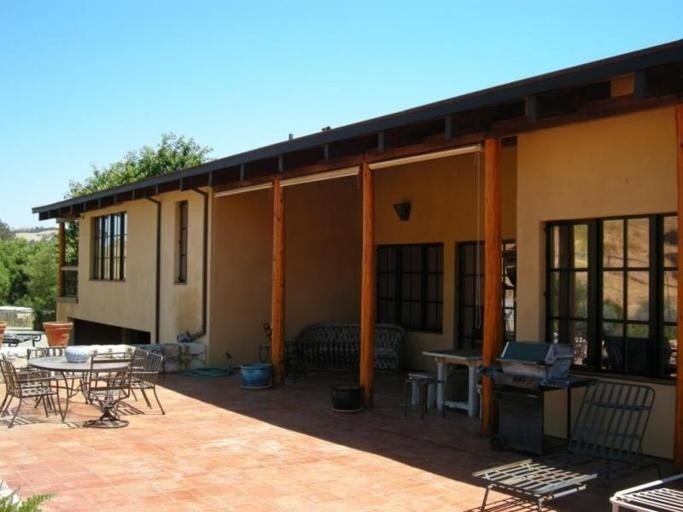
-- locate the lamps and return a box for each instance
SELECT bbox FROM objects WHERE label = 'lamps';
[392,203,412,223]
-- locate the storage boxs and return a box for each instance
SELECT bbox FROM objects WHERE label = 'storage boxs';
[496,337,574,377]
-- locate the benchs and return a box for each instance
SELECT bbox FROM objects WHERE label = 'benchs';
[277,317,404,378]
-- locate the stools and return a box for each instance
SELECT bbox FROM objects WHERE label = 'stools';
[398,377,447,420]
[406,370,441,408]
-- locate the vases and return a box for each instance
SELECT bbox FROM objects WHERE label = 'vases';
[39,320,74,349]
[239,361,272,391]
[327,384,365,414]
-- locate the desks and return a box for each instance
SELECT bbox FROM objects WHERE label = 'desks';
[422,346,483,421]
[487,370,596,459]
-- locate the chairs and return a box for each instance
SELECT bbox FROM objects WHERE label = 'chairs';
[0,342,168,430]
[470,375,659,512]
[607,474,683,511]
